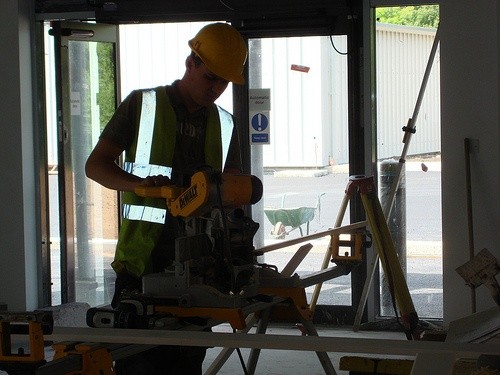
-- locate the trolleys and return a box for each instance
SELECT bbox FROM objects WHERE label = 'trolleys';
[263,192,326,240]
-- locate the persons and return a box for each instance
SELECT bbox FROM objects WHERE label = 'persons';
[85,23,249,375]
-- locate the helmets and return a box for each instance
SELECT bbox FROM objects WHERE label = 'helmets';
[188,22,248,85]
